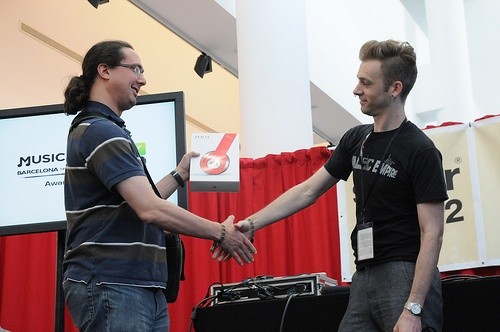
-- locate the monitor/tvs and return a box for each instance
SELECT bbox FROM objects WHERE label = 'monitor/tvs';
[0,91,187,236]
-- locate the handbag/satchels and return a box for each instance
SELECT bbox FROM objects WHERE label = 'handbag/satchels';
[67,110,186,304]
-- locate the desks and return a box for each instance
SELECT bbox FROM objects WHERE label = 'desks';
[193,276,500,332]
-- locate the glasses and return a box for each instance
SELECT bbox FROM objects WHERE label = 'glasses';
[107,63,144,75]
